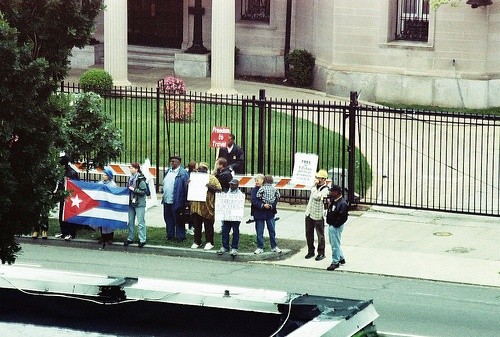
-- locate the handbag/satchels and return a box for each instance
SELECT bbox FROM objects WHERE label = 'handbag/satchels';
[137,178,151,196]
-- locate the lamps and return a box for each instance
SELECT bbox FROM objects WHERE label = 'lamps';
[466,0,493,9]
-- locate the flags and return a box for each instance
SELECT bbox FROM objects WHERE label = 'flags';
[62,178,130,231]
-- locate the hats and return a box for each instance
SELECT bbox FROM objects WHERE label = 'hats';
[316,170,328,179]
[328,185,341,193]
[198,162,209,171]
[228,179,239,185]
[103,169,114,179]
[131,163,140,170]
[169,156,181,163]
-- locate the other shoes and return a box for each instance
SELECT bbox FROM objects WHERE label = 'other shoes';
[274,217,279,221]
[246,219,255,223]
[305,253,315,259]
[186,227,195,236]
[315,253,325,261]
[203,242,214,251]
[326,263,339,271]
[166,237,186,242]
[339,259,345,265]
[32,230,72,240]
[191,243,202,249]
[97,237,145,248]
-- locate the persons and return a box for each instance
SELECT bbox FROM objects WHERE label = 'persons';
[32,206,49,239]
[185,162,221,250]
[160,156,189,243]
[304,170,330,261]
[97,168,117,244]
[55,156,78,240]
[219,134,245,194]
[212,157,233,193]
[217,180,242,255]
[246,174,283,255]
[322,185,349,271]
[124,163,151,248]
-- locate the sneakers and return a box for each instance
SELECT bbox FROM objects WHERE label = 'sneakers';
[217,246,230,254]
[270,246,282,254]
[254,247,263,254]
[229,249,238,255]
